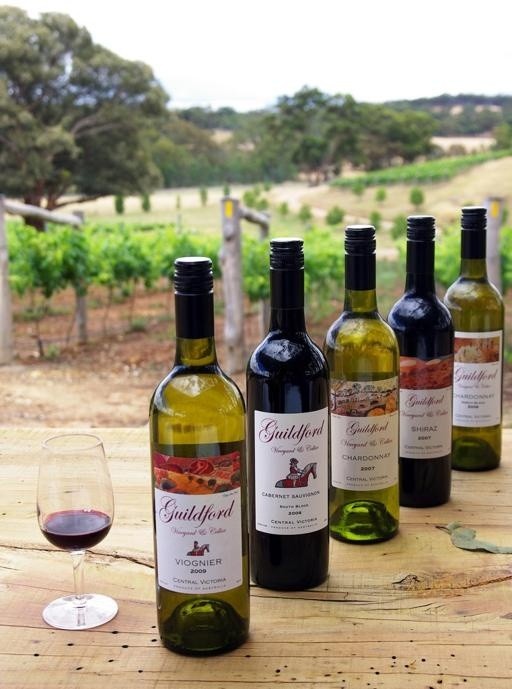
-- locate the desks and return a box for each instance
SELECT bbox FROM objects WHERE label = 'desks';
[2,424,512,685]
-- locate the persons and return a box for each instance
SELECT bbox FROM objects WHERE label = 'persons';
[192,541,200,552]
[287,458,303,487]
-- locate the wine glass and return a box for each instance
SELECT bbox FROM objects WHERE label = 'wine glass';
[35,433,119,632]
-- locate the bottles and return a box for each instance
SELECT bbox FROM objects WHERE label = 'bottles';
[244,236,334,593]
[321,223,401,543]
[146,256,250,656]
[384,213,456,510]
[441,206,504,474]
